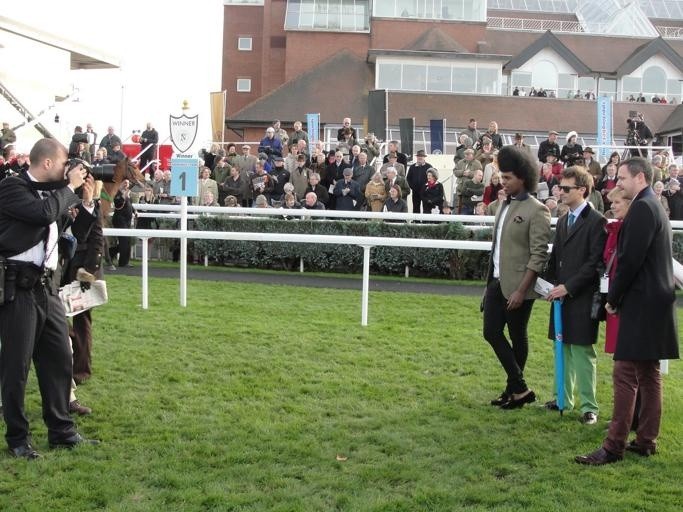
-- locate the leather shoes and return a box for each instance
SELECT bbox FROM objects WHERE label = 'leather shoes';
[575,446,624,464]
[8,445,42,458]
[625,441,658,456]
[491,391,510,405]
[501,391,537,410]
[69,399,91,414]
[49,432,101,448]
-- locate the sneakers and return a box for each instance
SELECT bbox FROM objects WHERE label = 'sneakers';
[583,412,598,424]
[104,264,117,271]
[545,399,558,410]
[120,264,135,269]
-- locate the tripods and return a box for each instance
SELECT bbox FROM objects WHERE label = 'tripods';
[621,130,643,160]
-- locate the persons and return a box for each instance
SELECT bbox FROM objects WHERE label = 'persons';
[597,163,618,212]
[479,144,505,216]
[551,164,570,218]
[600,151,620,177]
[55,160,104,383]
[99,147,107,159]
[318,118,367,221]
[587,176,604,215]
[68,141,83,159]
[79,142,91,165]
[406,150,434,223]
[574,156,680,465]
[306,162,318,220]
[575,147,603,189]
[0,153,30,181]
[560,131,583,168]
[512,86,682,105]
[385,140,399,223]
[108,177,138,270]
[419,168,444,223]
[65,334,90,414]
[136,181,159,243]
[479,144,551,410]
[653,151,670,156]
[649,156,680,220]
[551,144,560,164]
[539,164,609,424]
[538,144,551,208]
[0,138,101,460]
[479,120,560,144]
[367,148,385,221]
[159,119,307,220]
[399,162,406,223]
[108,142,127,163]
[138,124,159,181]
[624,111,652,158]
[91,150,109,167]
[84,124,97,161]
[0,123,17,155]
[680,164,683,220]
[601,182,643,431]
[72,126,87,142]
[452,119,479,225]
[99,126,122,156]
[3,145,16,153]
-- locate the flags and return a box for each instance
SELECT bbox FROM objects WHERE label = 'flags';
[430,119,443,155]
[399,118,413,162]
[597,97,611,156]
[306,114,318,162]
[367,90,385,148]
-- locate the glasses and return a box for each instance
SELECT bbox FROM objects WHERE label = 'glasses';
[558,184,584,194]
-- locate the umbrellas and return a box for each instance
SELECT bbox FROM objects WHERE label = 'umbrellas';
[553,280,565,418]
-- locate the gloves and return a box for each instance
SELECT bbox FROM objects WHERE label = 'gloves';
[80,282,90,292]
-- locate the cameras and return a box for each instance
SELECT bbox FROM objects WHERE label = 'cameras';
[66,159,117,183]
[626,110,641,130]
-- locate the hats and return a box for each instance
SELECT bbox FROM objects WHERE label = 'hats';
[415,150,427,157]
[242,145,251,149]
[365,133,378,140]
[482,139,492,145]
[426,168,438,180]
[274,157,284,161]
[385,152,399,158]
[582,147,596,155]
[439,201,454,209]
[256,195,267,205]
[565,131,578,144]
[463,148,474,155]
[548,130,559,137]
[343,168,354,176]
[465,137,474,146]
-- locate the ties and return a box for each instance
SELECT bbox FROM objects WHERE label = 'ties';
[40,193,49,272]
[568,213,576,225]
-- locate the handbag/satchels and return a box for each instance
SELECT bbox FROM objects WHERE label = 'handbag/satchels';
[590,292,608,320]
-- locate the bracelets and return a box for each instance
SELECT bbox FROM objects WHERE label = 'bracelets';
[82,200,94,208]
